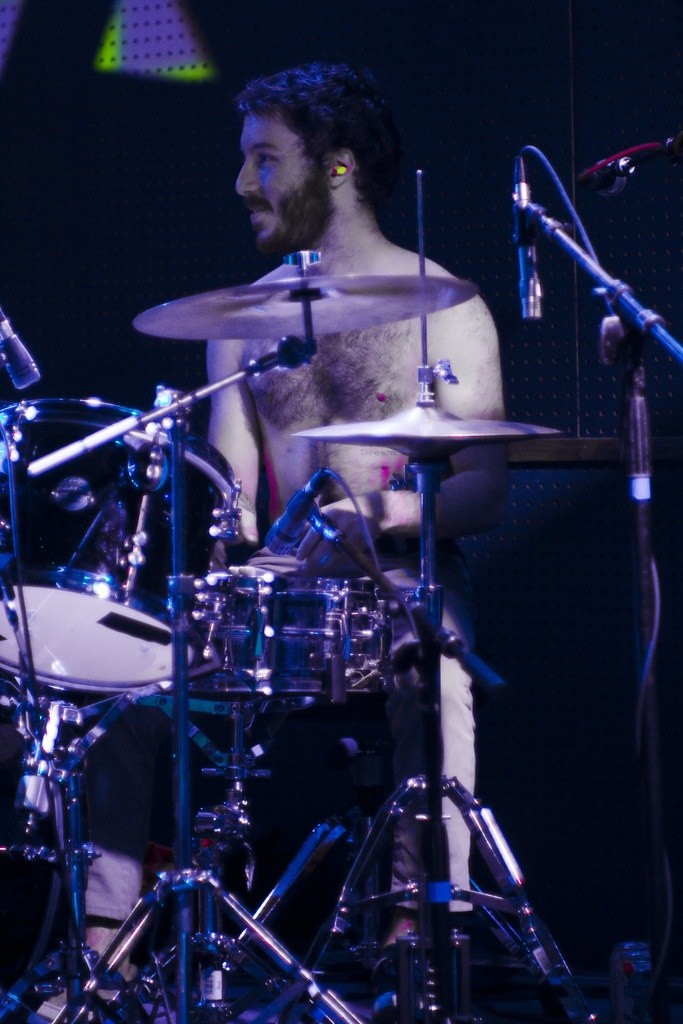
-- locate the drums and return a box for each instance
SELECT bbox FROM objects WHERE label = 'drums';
[0,396,241,693]
[178,566,389,696]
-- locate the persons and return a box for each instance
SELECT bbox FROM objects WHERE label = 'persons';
[26,57,503,1022]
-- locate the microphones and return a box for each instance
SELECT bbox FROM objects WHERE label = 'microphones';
[0,307,42,390]
[512,182,544,320]
[53,474,114,512]
[265,468,330,556]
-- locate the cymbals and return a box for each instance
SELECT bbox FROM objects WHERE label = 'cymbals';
[290,405,562,459]
[133,273,477,341]
